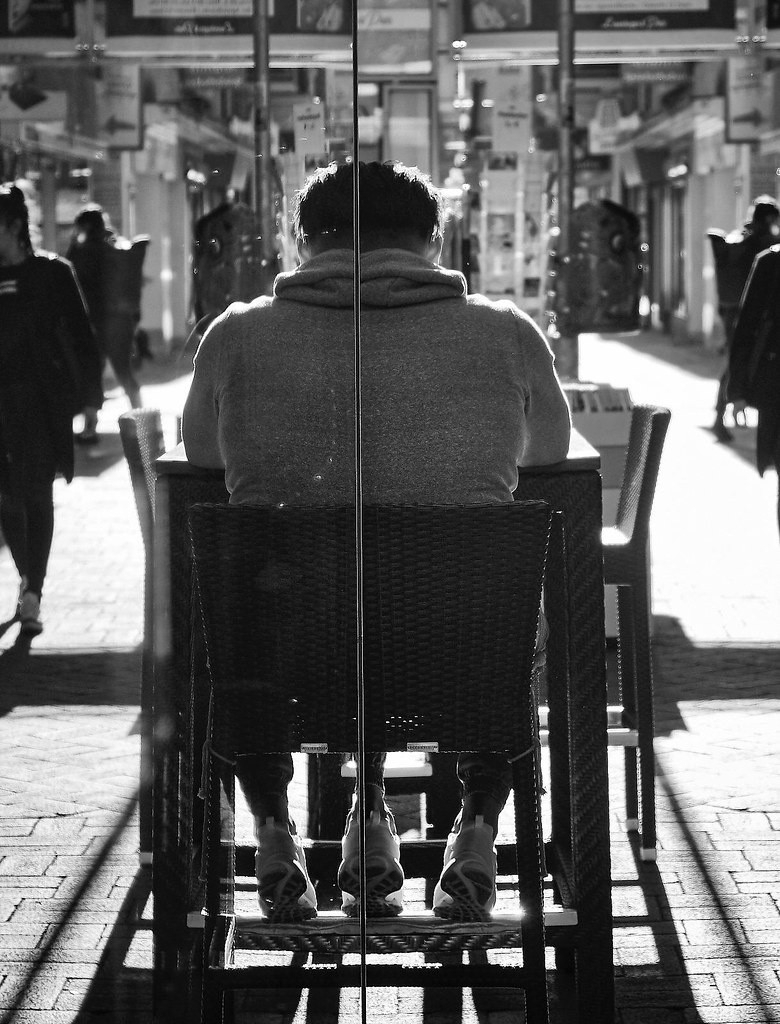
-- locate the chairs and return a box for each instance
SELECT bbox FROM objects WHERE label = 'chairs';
[537,403,673,854]
[121,406,169,855]
[184,493,556,1023]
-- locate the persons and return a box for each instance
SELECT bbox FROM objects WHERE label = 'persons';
[711,191,780,442]
[61,202,143,448]
[2,185,101,636]
[723,246,779,494]
[177,160,570,925]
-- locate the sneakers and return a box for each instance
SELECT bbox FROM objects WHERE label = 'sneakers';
[363,815,403,918]
[336,813,364,918]
[254,817,318,919]
[431,821,498,920]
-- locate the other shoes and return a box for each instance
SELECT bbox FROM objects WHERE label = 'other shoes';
[18,592,44,629]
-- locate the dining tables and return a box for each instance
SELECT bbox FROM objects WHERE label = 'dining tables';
[153,427,618,1024]
